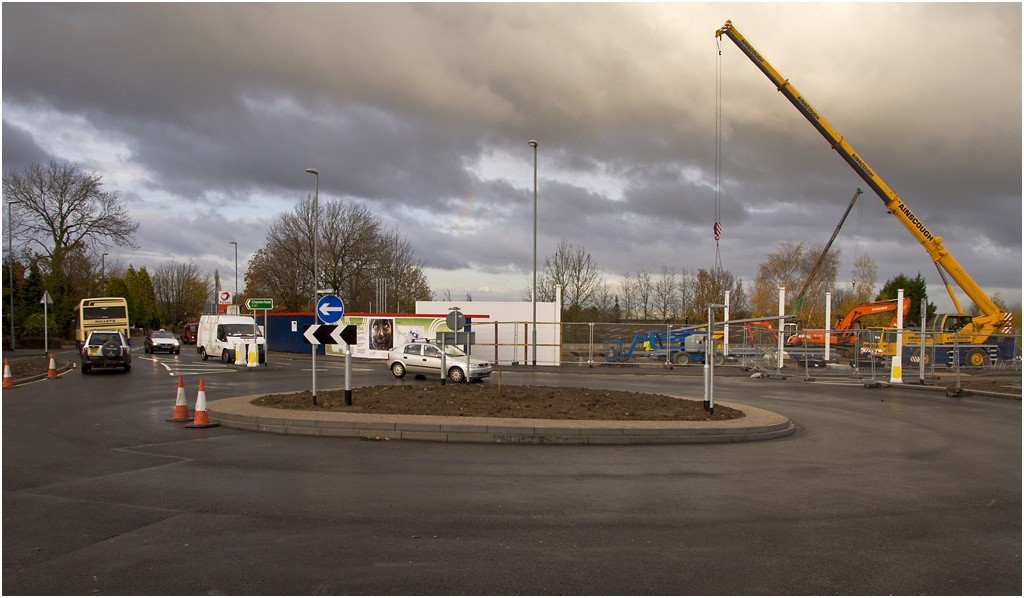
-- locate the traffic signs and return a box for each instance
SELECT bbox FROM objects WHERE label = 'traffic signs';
[244,298,274,311]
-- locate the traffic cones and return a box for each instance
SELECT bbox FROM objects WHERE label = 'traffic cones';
[184,379,223,429]
[2,357,16,389]
[48,352,58,379]
[102,253,108,297]
[165,376,194,422]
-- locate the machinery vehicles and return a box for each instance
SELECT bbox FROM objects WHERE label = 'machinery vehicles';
[714,19,1015,374]
[742,188,911,369]
[605,325,724,367]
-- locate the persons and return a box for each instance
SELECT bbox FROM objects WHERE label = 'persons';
[369,318,393,350]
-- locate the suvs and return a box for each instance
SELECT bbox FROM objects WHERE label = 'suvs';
[182,323,198,345]
[80,329,132,374]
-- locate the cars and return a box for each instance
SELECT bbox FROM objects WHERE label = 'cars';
[387,337,493,383]
[144,329,180,355]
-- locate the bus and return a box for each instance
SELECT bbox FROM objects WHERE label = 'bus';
[74,297,131,355]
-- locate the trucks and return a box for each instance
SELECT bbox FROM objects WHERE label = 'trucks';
[196,315,268,363]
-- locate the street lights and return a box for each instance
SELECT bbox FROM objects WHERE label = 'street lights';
[305,168,318,408]
[229,241,237,316]
[8,201,20,352]
[528,140,538,366]
[146,283,152,337]
